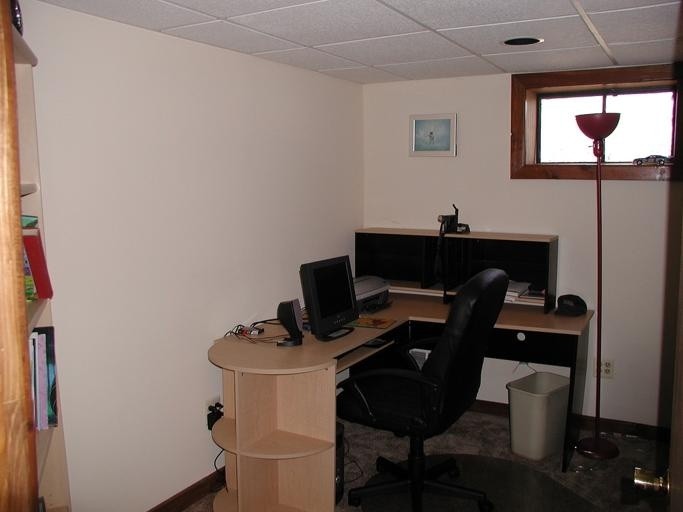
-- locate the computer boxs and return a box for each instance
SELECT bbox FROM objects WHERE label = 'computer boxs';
[336,422,345,506]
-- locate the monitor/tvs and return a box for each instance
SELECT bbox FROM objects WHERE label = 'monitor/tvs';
[299,255,359,342]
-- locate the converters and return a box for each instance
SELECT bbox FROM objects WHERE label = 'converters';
[208,410,222,430]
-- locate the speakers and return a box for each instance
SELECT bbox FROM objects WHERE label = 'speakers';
[276,298,303,347]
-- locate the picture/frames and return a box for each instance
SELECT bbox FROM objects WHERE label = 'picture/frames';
[407,112,458,159]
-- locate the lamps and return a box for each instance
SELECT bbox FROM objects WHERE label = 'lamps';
[576,109,627,464]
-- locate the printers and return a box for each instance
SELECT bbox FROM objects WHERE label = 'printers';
[353,275,393,314]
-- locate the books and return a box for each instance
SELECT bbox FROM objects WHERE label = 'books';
[21,226,60,430]
[504,279,545,307]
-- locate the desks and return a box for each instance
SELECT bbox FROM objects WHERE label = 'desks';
[204,293,595,511]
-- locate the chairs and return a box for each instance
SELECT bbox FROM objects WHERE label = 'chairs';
[336,256,515,511]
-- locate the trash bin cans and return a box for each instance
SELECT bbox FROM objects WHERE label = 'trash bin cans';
[506,372,571,461]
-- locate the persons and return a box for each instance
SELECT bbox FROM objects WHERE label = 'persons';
[428,132,434,144]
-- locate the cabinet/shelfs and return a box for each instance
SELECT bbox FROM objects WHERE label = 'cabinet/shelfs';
[0,0,76,512]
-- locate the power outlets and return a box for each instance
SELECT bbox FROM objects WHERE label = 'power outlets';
[593,356,615,380]
